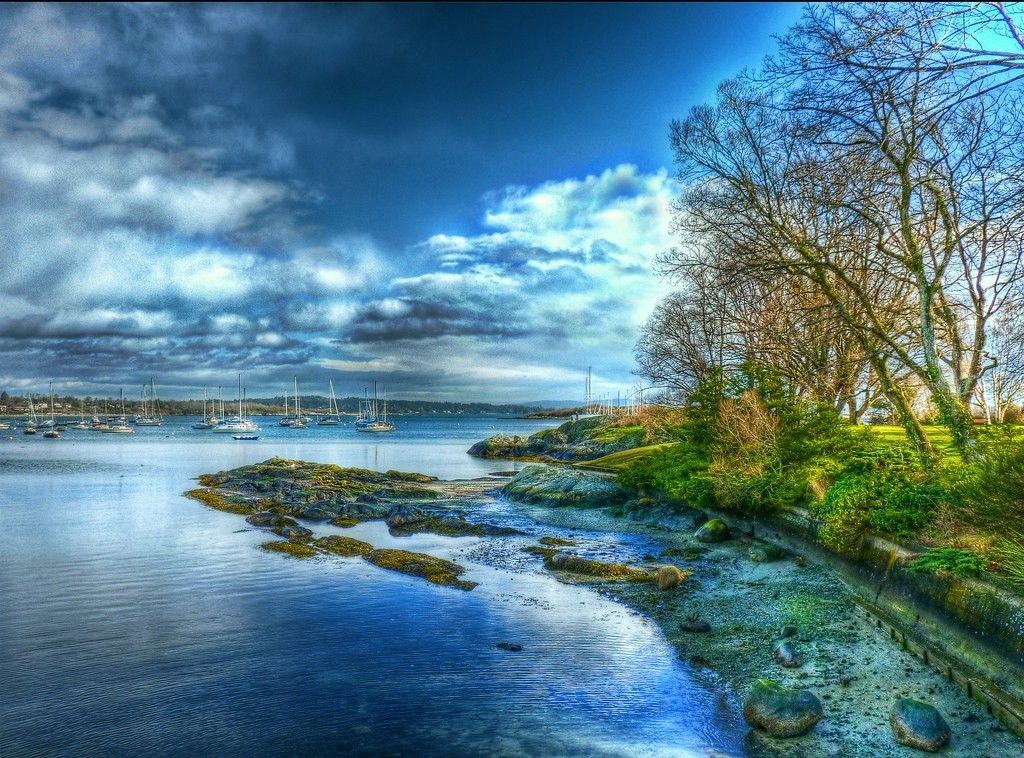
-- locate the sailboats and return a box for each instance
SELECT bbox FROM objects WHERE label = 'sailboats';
[280,376,313,427]
[355,382,395,431]
[192,373,258,432]
[16,380,134,439]
[316,379,341,424]
[135,378,164,426]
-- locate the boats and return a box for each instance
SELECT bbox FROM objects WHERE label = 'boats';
[232,436,259,440]
[0,425,10,429]
